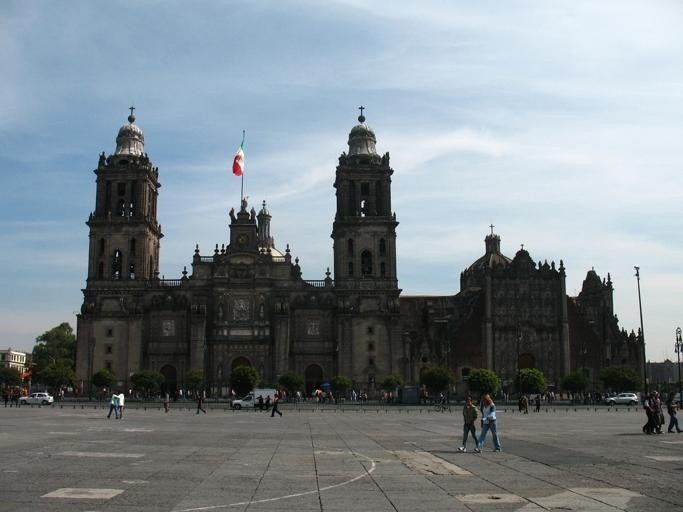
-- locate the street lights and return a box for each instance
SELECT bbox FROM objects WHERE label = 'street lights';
[674,326,683,409]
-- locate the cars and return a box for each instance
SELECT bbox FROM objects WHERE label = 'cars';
[15,391,54,406]
[605,392,639,406]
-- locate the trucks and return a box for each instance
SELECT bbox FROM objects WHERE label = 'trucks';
[230,388,279,410]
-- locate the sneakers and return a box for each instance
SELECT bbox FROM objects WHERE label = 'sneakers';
[640,425,682,434]
[457,445,501,453]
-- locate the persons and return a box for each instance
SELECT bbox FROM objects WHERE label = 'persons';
[637,389,682,435]
[194,394,206,415]
[278,384,392,406]
[0,384,64,409]
[163,391,169,413]
[438,394,446,410]
[517,389,616,415]
[269,393,282,417]
[475,393,502,453]
[255,395,265,413]
[106,391,119,420]
[456,396,481,454]
[70,383,238,402]
[263,395,272,410]
[111,391,125,420]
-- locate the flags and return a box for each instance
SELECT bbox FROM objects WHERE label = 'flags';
[230,139,246,178]
